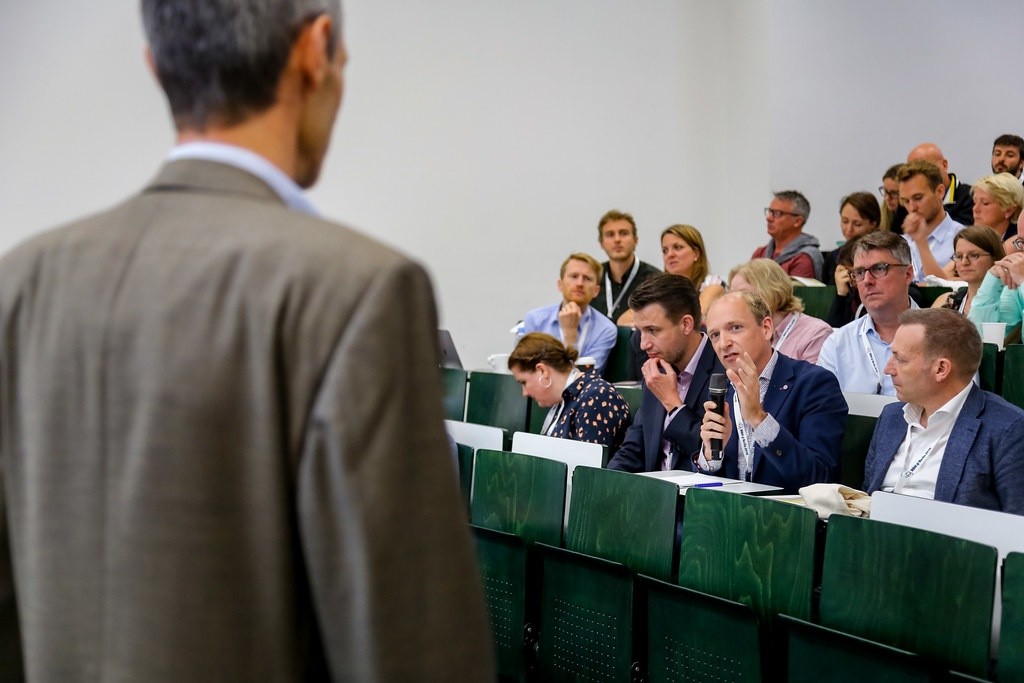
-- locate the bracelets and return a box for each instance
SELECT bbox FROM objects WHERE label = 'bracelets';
[668,406,678,417]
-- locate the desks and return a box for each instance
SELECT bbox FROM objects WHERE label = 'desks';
[512,431,608,542]
[634,470,784,500]
[444,419,509,503]
[869,490,1023,661]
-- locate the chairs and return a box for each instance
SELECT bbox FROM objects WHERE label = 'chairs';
[439,286,1023,683]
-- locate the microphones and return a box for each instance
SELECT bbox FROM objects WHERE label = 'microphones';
[709,373,728,459]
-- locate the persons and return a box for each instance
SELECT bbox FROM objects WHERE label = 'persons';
[507,133,1024,517]
[0,0,491,683]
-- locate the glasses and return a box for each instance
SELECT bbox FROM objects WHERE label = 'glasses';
[763,207,798,219]
[848,262,907,281]
[950,252,992,262]
[1012,237,1024,251]
[879,186,898,201]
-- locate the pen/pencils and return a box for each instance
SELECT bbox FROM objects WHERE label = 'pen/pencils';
[694,483,723,487]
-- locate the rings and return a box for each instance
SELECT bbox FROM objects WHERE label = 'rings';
[1004,269,1007,271]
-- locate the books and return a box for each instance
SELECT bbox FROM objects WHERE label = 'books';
[791,276,826,288]
[612,381,642,389]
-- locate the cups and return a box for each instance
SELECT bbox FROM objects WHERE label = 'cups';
[575,357,596,373]
[982,322,1008,350]
[487,354,510,374]
